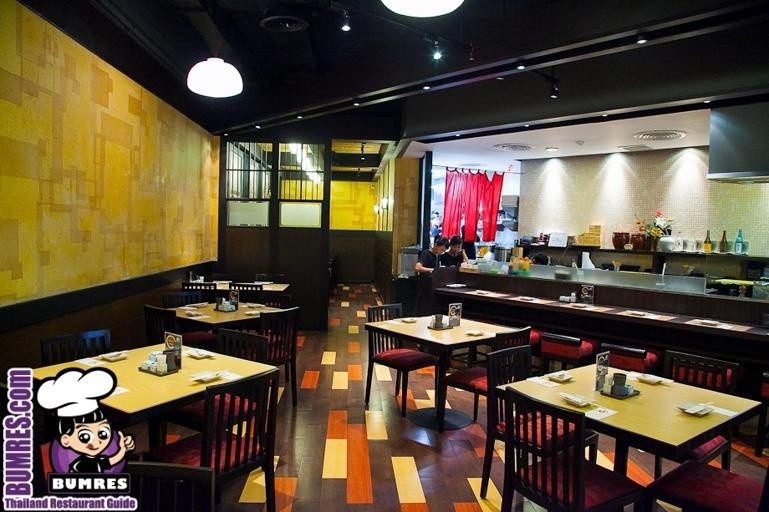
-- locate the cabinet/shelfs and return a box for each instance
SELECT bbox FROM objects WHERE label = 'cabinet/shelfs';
[457,264,769,321]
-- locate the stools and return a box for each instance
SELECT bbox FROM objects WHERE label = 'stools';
[601,343,662,373]
[674,358,731,387]
[504,328,542,346]
[541,332,600,370]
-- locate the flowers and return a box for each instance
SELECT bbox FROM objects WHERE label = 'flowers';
[634,210,673,237]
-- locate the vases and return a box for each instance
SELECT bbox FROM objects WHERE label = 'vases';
[659,235,676,251]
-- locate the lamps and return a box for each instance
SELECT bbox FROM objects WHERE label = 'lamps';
[352,95,360,106]
[374,204,379,229]
[290,143,323,185]
[186,34,243,98]
[340,8,352,31]
[636,29,647,43]
[549,68,560,99]
[517,61,525,69]
[223,130,229,136]
[433,41,442,60]
[255,122,262,129]
[382,196,388,229]
[297,108,303,118]
[360,143,366,159]
[423,83,431,89]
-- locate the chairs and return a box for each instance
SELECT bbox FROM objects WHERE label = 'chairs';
[0,383,53,495]
[755,370,769,457]
[142,368,280,512]
[654,349,740,481]
[647,458,769,512]
[148,328,268,471]
[501,386,646,512]
[439,326,532,434]
[41,329,111,363]
[182,282,217,295]
[143,304,219,345]
[161,293,202,308]
[229,283,263,296]
[236,306,299,407]
[365,304,439,417]
[481,346,598,500]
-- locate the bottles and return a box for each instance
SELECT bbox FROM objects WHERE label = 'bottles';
[513,256,530,275]
[508,260,513,274]
[719,231,729,252]
[734,229,744,253]
[703,230,711,252]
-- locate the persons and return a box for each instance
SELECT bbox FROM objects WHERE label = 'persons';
[544,235,548,241]
[539,232,543,240]
[483,244,496,260]
[529,254,548,265]
[461,225,480,259]
[415,235,456,275]
[430,209,440,248]
[442,236,468,267]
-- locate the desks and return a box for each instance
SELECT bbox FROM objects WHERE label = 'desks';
[434,286,769,341]
[185,284,290,292]
[495,363,763,476]
[33,343,277,455]
[166,302,280,334]
[364,315,520,430]
